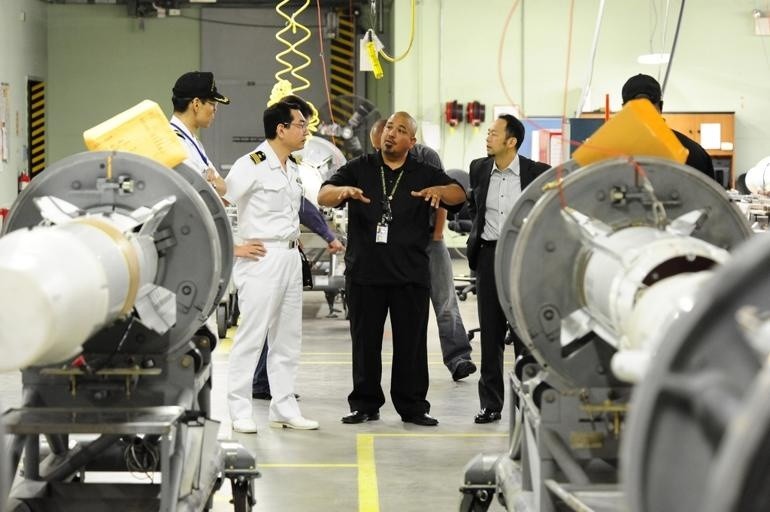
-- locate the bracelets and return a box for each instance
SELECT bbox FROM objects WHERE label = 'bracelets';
[211,182,218,190]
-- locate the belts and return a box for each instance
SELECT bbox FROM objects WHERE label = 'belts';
[288,240,297,248]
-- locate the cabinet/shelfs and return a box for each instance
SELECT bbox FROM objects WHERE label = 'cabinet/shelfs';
[579,111,737,190]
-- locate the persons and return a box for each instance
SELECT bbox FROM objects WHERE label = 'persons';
[321,112,449,425]
[622,73,715,179]
[369,120,477,380]
[167,70,231,199]
[223,100,320,432]
[466,114,554,423]
[250,96,347,400]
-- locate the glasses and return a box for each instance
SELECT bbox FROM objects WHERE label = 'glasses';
[283,122,308,129]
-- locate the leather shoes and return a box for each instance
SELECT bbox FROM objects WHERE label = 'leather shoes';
[402,413,438,426]
[252,392,299,400]
[270,415,319,430]
[232,418,257,433]
[453,362,476,381]
[341,411,379,424]
[475,407,501,423]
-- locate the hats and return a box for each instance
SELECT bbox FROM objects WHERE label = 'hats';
[172,71,230,105]
[622,73,661,104]
